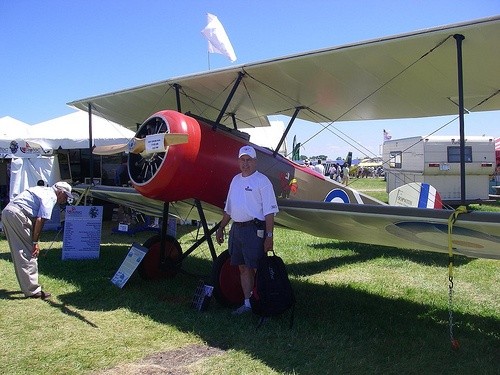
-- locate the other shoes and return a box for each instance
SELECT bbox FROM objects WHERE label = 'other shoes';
[237,304,252,315]
[32,293,51,298]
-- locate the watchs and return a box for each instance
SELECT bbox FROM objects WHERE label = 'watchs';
[265,232,273,237]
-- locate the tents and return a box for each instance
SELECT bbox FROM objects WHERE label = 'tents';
[0,110,137,205]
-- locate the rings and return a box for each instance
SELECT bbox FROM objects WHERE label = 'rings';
[220,238,222,239]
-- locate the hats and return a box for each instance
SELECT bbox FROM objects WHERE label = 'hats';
[239,146,256,159]
[55,182,74,198]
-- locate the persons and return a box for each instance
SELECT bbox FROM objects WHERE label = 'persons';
[342,163,350,186]
[216,146,280,315]
[335,163,341,181]
[357,166,363,179]
[2,182,74,299]
[305,159,325,175]
[329,164,336,179]
[365,166,381,177]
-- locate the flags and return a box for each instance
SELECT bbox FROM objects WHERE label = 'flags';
[202,13,237,62]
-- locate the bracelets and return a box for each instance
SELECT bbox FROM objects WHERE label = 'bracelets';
[219,226,224,229]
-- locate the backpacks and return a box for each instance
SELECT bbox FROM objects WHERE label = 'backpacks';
[249,251,296,317]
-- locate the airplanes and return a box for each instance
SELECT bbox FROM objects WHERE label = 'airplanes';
[66,17,500,302]
